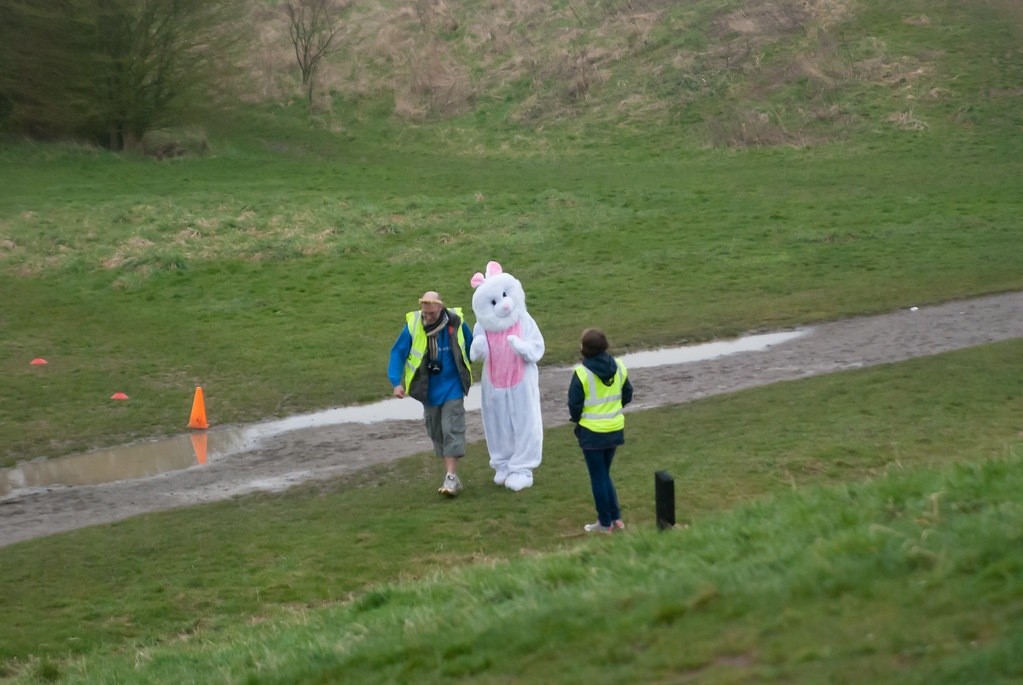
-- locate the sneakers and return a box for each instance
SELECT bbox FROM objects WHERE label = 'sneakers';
[438,472,465,495]
[584,519,614,533]
[596,518,625,530]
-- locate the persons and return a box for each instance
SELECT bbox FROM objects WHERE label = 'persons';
[566,327,634,535]
[386,292,476,498]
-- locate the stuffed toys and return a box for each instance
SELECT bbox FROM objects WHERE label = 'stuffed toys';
[469,260,545,491]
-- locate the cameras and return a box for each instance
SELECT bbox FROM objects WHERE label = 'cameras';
[425,359,443,375]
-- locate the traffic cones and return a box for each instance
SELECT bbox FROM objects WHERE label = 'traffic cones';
[189,431,208,465]
[186,386,210,429]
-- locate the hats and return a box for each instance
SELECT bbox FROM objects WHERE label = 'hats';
[419,292,444,306]
